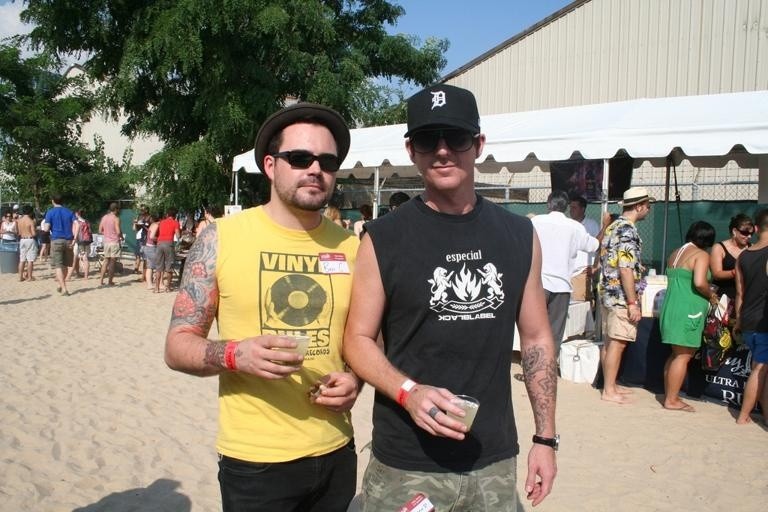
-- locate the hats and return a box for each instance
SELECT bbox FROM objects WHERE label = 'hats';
[255,103,350,178]
[617,187,656,206]
[404,85,481,138]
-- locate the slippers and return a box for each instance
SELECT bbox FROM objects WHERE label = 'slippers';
[665,404,695,413]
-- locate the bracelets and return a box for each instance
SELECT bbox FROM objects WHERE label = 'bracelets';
[731,269,736,278]
[531,434,560,450]
[398,379,416,408]
[225,340,237,368]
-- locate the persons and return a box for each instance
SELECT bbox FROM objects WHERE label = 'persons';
[354,204,372,240]
[709,213,756,301]
[594,186,653,404]
[389,190,410,211]
[0,193,80,297]
[98,202,124,287]
[324,206,342,228]
[514,189,600,386]
[133,203,182,293]
[567,198,599,272]
[658,220,716,412]
[733,207,768,430]
[195,205,223,237]
[338,83,560,510]
[65,209,91,281]
[163,100,365,511]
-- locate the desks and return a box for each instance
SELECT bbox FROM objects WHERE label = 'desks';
[616,317,762,414]
[513,300,596,352]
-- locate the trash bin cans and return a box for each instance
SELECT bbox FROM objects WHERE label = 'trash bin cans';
[0,243,19,273]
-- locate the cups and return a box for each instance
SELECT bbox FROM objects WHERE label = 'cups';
[648,268,656,280]
[445,393,482,433]
[276,335,311,370]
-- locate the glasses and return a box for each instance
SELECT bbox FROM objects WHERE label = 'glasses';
[738,229,754,237]
[411,128,474,153]
[273,150,342,173]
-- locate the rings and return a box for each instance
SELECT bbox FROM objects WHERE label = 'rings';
[428,407,440,418]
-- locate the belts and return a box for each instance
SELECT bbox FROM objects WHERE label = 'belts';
[20,237,32,239]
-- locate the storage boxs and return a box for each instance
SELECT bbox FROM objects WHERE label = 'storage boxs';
[571,274,592,302]
[641,274,668,317]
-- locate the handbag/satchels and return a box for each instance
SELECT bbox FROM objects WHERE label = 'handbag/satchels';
[704,314,731,349]
[702,348,763,415]
